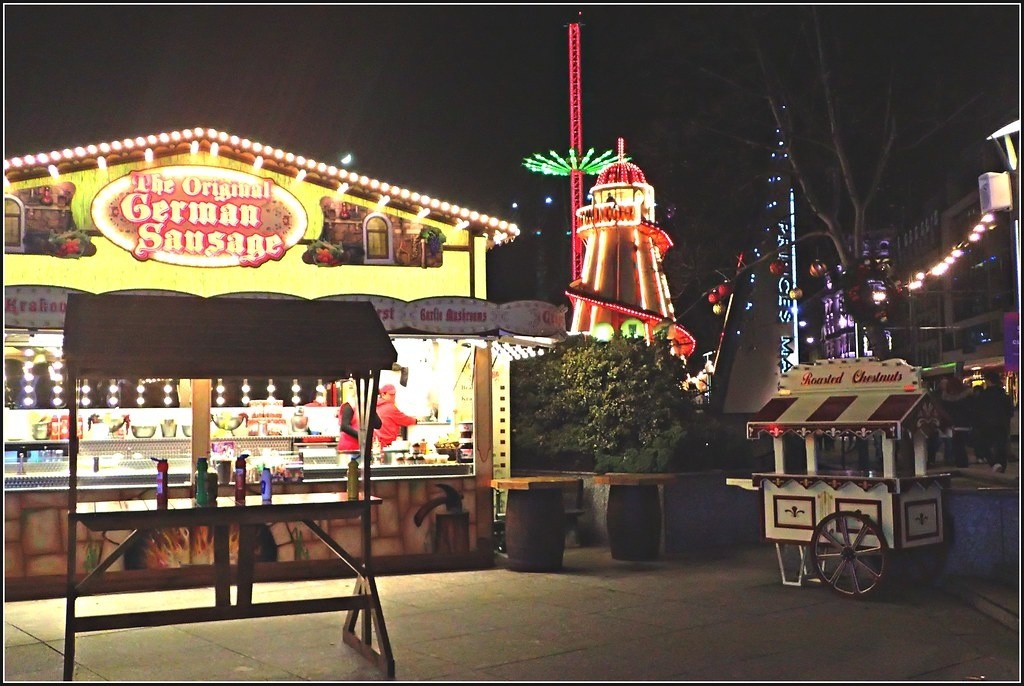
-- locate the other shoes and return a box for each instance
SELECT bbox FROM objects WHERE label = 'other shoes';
[978,457,988,463]
[992,463,1002,472]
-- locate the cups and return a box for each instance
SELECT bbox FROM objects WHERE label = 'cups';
[216,461,231,484]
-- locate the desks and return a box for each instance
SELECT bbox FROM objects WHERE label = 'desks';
[63,490,397,682]
[490,477,584,572]
[594,473,676,560]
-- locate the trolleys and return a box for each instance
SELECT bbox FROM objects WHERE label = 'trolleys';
[726,356,954,600]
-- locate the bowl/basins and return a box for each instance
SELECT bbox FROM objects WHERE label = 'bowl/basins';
[131,426,157,438]
[182,426,192,437]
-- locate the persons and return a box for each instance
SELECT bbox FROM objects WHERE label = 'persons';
[922,381,940,465]
[372,385,434,449]
[337,386,359,450]
[976,372,1014,472]
[942,380,974,468]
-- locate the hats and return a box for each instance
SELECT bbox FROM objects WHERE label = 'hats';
[379,384,396,394]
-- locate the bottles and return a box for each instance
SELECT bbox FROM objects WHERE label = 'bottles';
[236,454,249,500]
[420,438,426,453]
[347,457,360,498]
[261,468,272,501]
[150,457,168,503]
[196,457,209,503]
